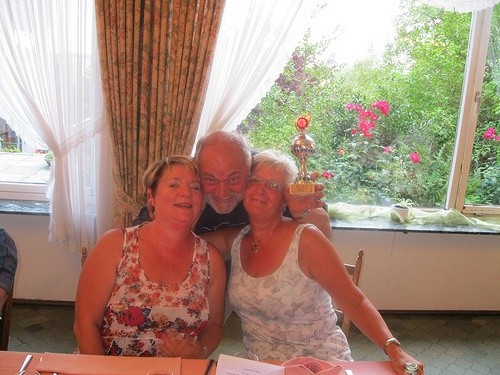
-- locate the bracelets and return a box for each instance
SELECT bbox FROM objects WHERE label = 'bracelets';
[383,338,400,355]
[292,209,311,221]
[199,341,210,359]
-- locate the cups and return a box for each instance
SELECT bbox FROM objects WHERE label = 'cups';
[146,367,174,375]
[235,352,259,362]
[16,369,41,375]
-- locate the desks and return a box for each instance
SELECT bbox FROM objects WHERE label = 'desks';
[0,350,399,375]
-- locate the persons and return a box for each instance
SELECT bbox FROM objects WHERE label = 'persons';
[0,228,18,319]
[72,155,227,361]
[199,147,423,375]
[131,131,330,234]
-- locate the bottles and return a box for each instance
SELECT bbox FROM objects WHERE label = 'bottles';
[405,361,418,375]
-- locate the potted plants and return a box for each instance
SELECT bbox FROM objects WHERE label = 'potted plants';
[388,197,421,224]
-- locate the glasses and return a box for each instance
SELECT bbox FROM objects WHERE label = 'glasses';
[248,175,282,192]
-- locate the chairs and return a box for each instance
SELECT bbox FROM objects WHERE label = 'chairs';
[333,249,364,339]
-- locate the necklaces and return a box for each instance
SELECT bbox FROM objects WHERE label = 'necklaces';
[248,217,284,253]
